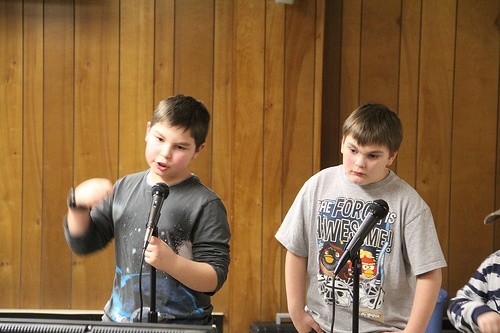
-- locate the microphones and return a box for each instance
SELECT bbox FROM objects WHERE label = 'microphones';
[334,199,389,277]
[142,182,169,258]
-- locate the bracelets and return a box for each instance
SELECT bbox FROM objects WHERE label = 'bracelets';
[67,187,94,211]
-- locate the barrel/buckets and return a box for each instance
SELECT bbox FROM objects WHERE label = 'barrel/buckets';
[425,288,449,333]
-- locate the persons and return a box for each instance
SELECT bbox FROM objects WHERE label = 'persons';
[275,102,450,333]
[445,248,500,333]
[60,92,231,326]
